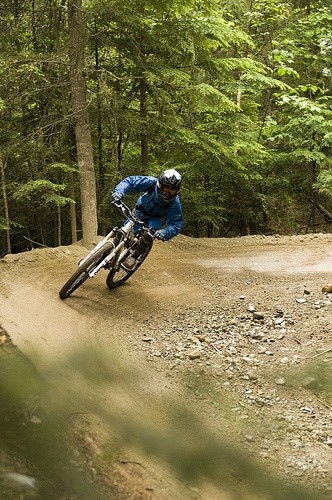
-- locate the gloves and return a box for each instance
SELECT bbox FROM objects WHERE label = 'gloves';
[154,229,167,240]
[111,191,124,205]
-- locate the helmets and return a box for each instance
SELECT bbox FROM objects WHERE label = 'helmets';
[157,169,182,205]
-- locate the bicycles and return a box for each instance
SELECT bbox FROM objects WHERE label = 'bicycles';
[58,192,165,299]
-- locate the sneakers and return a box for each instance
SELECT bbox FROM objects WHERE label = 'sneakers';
[123,256,136,269]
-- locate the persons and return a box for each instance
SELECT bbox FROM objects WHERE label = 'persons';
[111,168,184,268]
